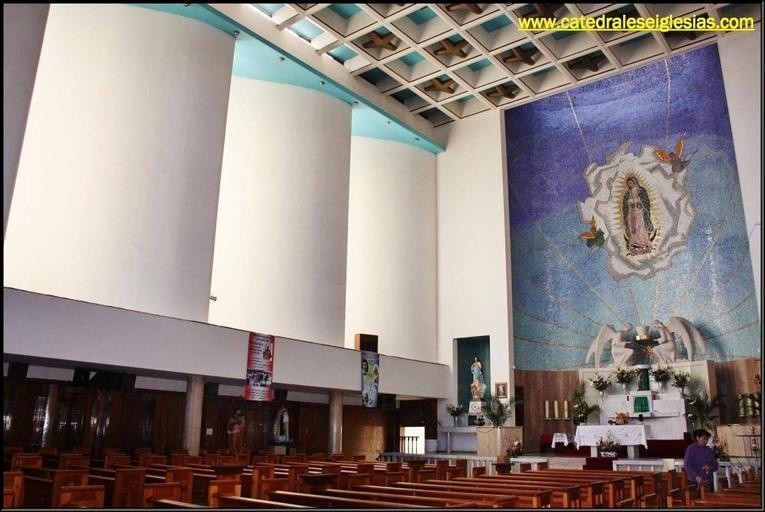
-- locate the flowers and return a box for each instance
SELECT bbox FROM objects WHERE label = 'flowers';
[506,441,524,457]
[588,367,635,392]
[445,403,464,415]
[649,366,689,388]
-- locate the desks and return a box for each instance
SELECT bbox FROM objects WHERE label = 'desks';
[441,426,480,455]
[576,424,647,458]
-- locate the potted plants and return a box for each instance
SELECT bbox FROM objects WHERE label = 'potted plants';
[596,431,621,458]
[419,403,441,452]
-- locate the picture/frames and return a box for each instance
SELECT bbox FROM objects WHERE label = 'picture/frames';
[495,382,508,398]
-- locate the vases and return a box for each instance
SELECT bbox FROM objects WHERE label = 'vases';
[453,416,460,426]
[657,381,683,400]
[599,383,627,404]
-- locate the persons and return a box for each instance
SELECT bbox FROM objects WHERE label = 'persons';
[470,356,484,399]
[369,363,379,389]
[226,407,247,451]
[263,345,272,360]
[624,176,656,257]
[684,428,720,491]
[646,318,678,365]
[361,358,369,376]
[610,321,635,367]
